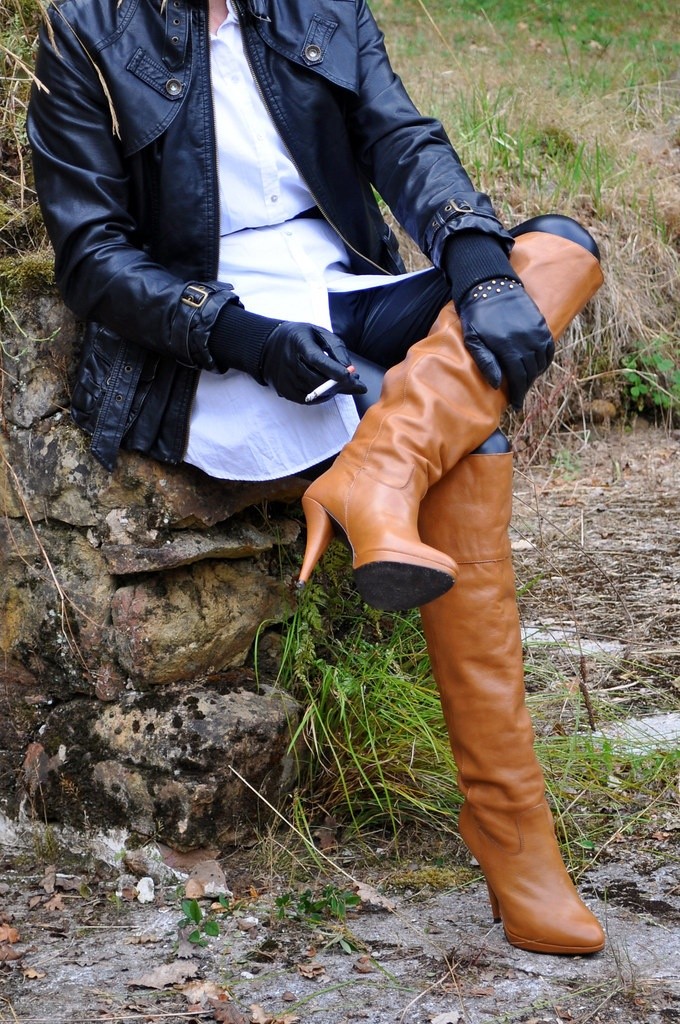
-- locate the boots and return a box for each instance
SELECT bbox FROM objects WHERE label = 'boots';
[403,451,605,954]
[294,231,603,610]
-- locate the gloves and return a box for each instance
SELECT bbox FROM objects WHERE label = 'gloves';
[455,277,555,411]
[259,322,368,406]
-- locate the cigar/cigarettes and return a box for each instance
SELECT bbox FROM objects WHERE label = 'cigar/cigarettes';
[305,366,354,402]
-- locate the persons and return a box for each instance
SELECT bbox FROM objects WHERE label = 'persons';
[26,0,610,956]
